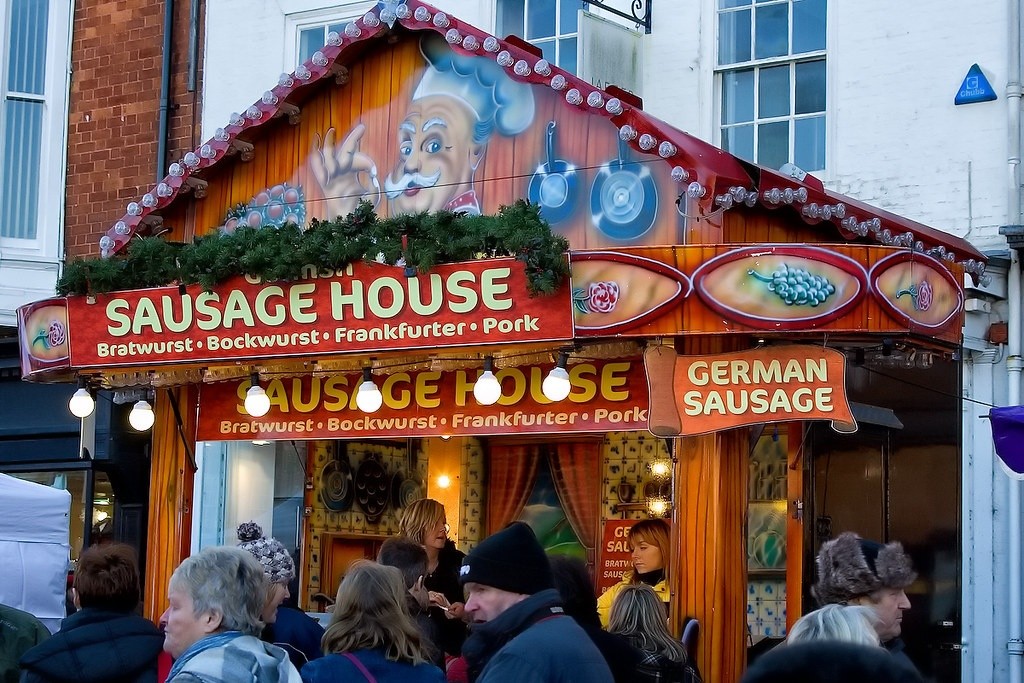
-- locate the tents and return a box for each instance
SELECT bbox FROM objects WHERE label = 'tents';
[0,472,53,628]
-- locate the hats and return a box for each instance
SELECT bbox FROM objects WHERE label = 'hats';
[459,523,554,596]
[811,532,913,606]
[237,522,296,584]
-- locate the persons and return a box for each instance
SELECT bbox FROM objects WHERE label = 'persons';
[606,582,703,683]
[546,553,643,683]
[811,532,921,677]
[459,521,614,683]
[236,519,296,643]
[596,518,671,631]
[270,582,326,671]
[377,535,446,675]
[787,602,892,656]
[18,540,176,683]
[160,545,303,683]
[299,557,448,683]
[396,498,466,657]
[0,603,51,683]
[738,640,924,683]
